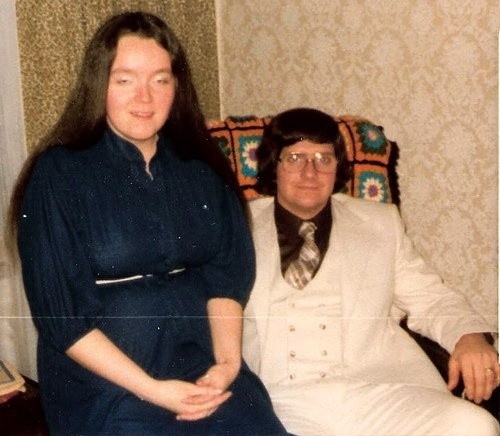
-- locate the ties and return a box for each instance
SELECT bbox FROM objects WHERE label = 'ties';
[286,221,318,290]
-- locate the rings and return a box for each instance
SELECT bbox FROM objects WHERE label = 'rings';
[485,368,494,375]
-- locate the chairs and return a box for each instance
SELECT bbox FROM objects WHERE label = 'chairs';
[207,114,495,399]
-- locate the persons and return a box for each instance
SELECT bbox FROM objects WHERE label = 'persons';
[239,108,500,436]
[5,11,298,435]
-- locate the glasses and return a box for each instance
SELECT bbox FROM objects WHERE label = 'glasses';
[281,152,337,175]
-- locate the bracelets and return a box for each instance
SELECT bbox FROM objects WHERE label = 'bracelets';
[139,374,151,403]
